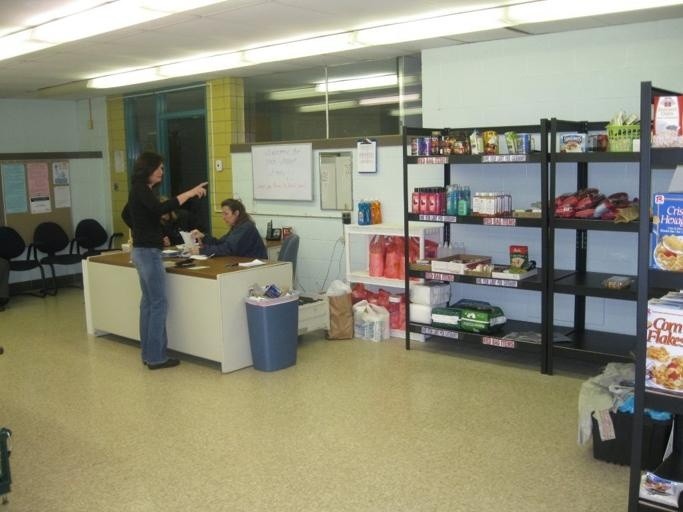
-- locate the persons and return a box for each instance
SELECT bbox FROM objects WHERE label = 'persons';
[189,199,267,260]
[158,196,185,247]
[121,153,209,370]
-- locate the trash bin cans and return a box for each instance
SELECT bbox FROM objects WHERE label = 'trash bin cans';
[245,288,300,372]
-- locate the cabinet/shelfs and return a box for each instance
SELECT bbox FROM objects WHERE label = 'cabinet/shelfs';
[627,80,683,512]
[401,118,586,374]
[545,118,680,376]
[343,220,444,343]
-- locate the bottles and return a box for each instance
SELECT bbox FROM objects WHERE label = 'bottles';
[430,127,470,155]
[357,199,382,226]
[436,241,466,259]
[412,183,514,217]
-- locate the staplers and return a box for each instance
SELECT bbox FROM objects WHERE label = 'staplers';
[175,258,196,268]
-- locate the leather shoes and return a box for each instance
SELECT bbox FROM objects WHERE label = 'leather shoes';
[143,359,179,369]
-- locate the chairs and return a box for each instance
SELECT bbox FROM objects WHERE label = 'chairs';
[0,218,124,298]
[278,234,299,281]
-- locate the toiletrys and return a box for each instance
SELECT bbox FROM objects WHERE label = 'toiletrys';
[445,184,470,217]
[357,198,382,225]
[411,186,446,215]
[472,191,512,218]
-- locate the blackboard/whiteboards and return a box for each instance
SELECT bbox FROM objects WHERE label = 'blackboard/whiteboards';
[251,142,313,201]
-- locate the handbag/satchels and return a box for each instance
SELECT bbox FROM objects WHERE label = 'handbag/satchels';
[320,291,353,340]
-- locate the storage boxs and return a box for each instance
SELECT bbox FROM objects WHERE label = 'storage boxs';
[576,362,673,472]
[409,303,448,325]
[410,282,450,305]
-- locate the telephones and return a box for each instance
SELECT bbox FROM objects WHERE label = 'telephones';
[266,220,282,240]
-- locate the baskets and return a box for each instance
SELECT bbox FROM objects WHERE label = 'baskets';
[605,124,641,152]
